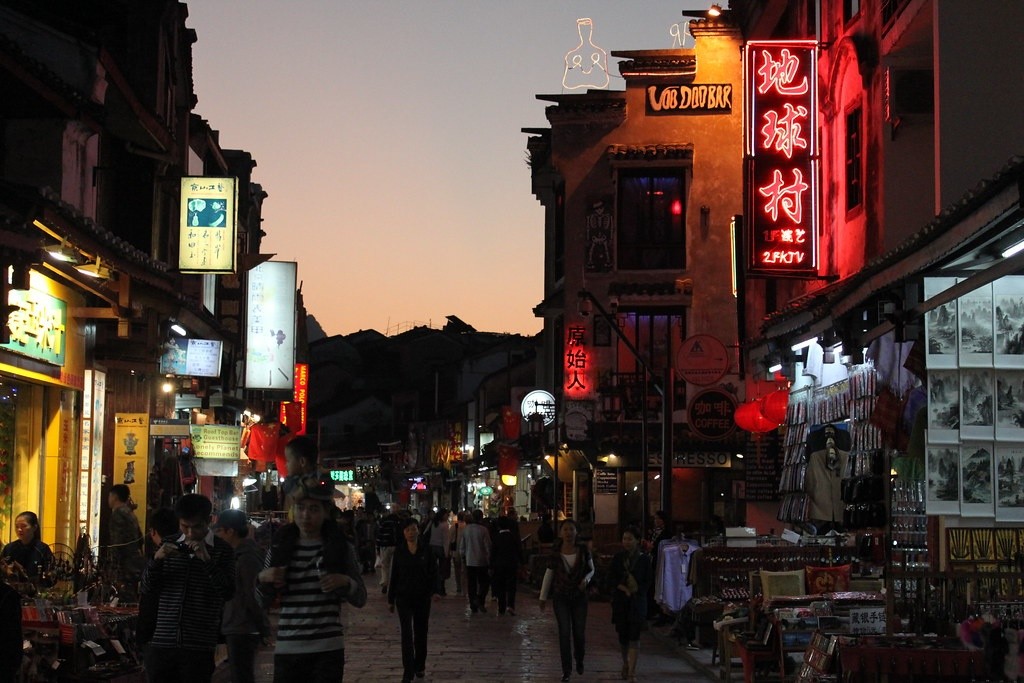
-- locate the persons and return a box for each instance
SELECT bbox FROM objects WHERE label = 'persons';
[539,519,596,683]
[641,510,673,563]
[106,434,553,683]
[0,511,57,585]
[388,517,437,683]
[604,525,654,683]
[706,514,725,540]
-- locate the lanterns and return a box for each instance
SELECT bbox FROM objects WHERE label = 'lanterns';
[733,388,790,458]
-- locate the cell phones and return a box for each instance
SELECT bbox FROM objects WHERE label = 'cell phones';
[173,542,194,554]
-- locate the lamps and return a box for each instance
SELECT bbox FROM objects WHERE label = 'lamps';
[44,238,83,263]
[76,255,112,279]
[761,344,784,374]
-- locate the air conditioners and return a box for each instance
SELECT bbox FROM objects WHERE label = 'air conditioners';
[884,64,930,122]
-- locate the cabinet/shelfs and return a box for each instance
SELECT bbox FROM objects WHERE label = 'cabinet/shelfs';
[775,608,848,673]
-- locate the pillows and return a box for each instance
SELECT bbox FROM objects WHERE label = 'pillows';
[759,569,805,601]
[806,565,852,594]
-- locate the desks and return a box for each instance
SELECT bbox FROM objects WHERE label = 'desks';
[836,634,985,683]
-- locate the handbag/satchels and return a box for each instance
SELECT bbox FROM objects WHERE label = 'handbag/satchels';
[622,576,643,610]
[254,575,276,609]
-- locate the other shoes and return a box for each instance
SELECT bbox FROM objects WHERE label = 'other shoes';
[629,674,638,683]
[416,670,424,678]
[323,592,341,614]
[402,676,413,683]
[496,611,504,616]
[507,607,516,614]
[480,606,487,612]
[621,665,629,680]
[561,671,571,682]
[472,610,478,615]
[576,659,584,675]
[268,557,287,587]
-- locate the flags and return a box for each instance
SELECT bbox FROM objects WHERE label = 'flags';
[240,420,288,478]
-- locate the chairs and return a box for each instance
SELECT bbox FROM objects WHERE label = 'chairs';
[532,539,554,552]
[601,543,622,564]
[718,625,770,683]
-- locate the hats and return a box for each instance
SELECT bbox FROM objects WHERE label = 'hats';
[290,479,331,501]
[214,509,248,528]
